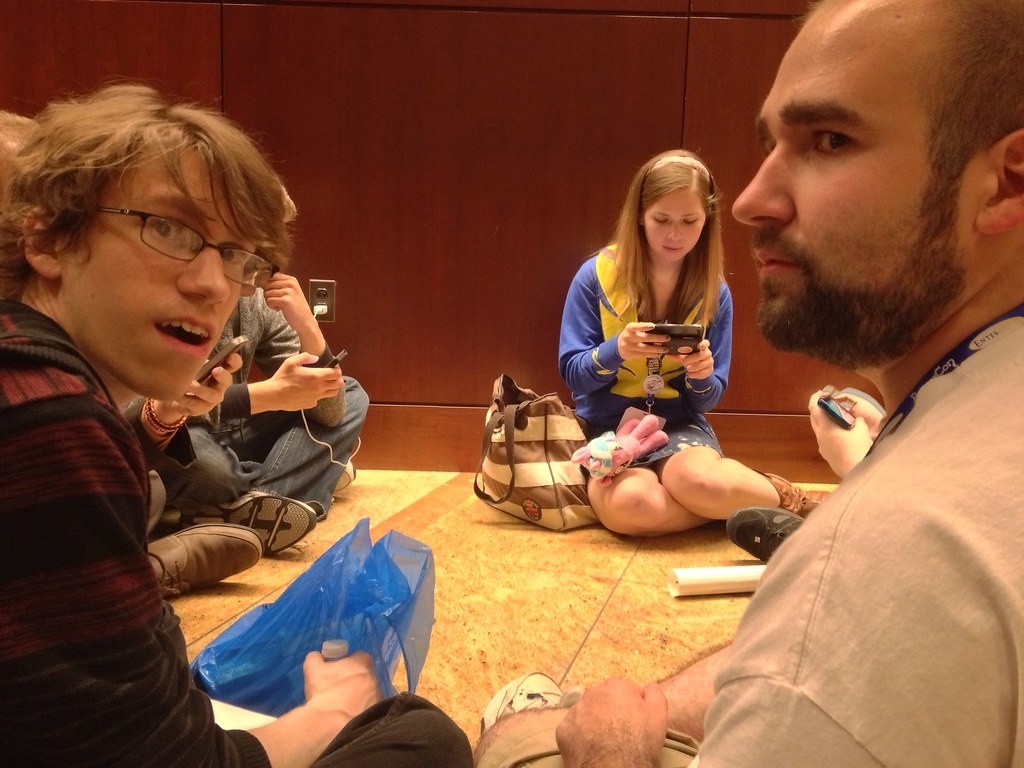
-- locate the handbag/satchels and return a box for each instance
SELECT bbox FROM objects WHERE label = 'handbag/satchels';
[472,374,599,531]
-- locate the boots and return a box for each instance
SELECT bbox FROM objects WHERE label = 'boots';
[752,467,830,515]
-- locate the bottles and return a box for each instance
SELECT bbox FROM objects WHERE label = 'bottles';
[322,639,348,663]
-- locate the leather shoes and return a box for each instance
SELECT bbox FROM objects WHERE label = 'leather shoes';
[147,523,263,600]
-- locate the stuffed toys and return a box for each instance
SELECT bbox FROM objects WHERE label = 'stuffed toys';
[570,414,670,487]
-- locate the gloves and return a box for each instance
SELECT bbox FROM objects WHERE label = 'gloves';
[570,414,666,486]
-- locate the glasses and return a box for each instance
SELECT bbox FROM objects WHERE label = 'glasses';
[88,205,280,288]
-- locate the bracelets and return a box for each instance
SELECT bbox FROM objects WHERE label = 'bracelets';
[144,395,188,433]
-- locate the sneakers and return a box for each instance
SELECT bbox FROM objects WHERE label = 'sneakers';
[481,670,584,734]
[177,489,317,557]
[726,506,804,563]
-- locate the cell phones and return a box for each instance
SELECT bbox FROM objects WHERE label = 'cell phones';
[818,395,856,429]
[324,350,348,368]
[645,324,705,355]
[195,335,249,383]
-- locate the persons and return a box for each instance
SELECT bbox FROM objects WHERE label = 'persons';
[1,84,469,768]
[559,149,833,541]
[725,385,887,564]
[1,112,369,599]
[471,0,1024,767]
